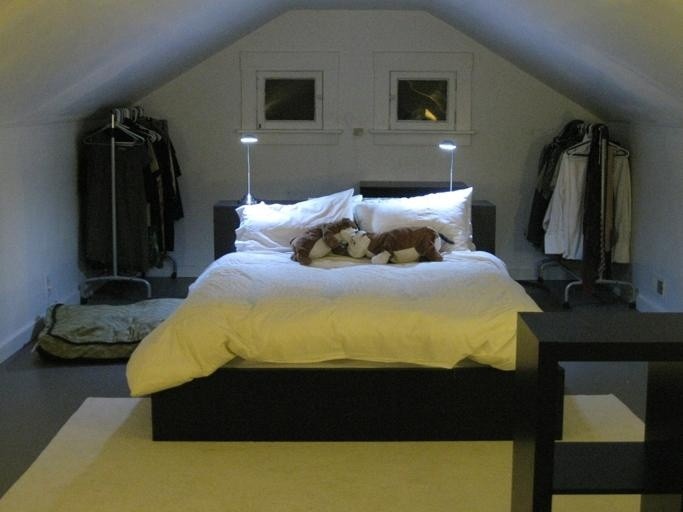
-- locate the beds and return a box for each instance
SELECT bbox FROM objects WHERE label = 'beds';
[126,183,564,441]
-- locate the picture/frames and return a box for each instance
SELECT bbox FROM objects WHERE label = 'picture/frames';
[240,50,341,144]
[369,51,471,146]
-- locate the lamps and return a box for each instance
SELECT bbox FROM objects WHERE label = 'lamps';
[237,134,262,204]
[439,139,457,191]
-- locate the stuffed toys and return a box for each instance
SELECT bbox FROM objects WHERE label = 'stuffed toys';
[347,226,456,266]
[290,217,358,265]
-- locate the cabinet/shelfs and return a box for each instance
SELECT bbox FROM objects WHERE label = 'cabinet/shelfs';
[511,311,683,512]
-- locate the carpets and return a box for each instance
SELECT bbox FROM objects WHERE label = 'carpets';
[0,393,646,512]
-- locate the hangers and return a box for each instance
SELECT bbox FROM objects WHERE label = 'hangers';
[549,117,631,163]
[79,104,167,146]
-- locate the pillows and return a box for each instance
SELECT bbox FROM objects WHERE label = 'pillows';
[30,296,183,358]
[232,188,476,253]
